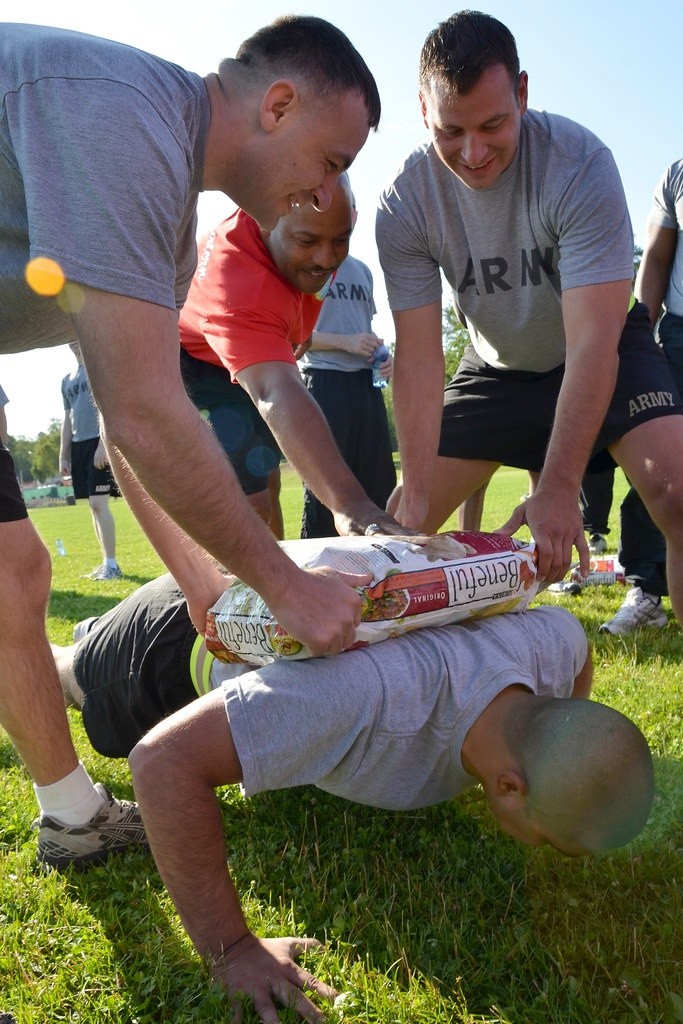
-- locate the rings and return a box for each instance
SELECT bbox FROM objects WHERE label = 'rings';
[364,521,381,538]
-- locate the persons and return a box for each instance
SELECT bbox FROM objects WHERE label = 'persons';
[0,12,375,866]
[456,468,585,599]
[382,7,683,637]
[597,153,683,643]
[1,386,10,446]
[580,438,622,554]
[58,340,124,583]
[42,567,659,1024]
[178,166,435,556]
[293,190,399,536]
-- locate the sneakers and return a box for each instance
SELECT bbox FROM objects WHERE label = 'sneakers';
[597,586,668,638]
[547,579,582,598]
[79,565,102,579]
[586,533,608,554]
[29,782,149,873]
[93,564,122,582]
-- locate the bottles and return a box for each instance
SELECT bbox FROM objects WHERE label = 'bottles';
[56,538,65,557]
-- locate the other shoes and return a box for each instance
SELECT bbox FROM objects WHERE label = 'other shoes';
[72,616,100,644]
